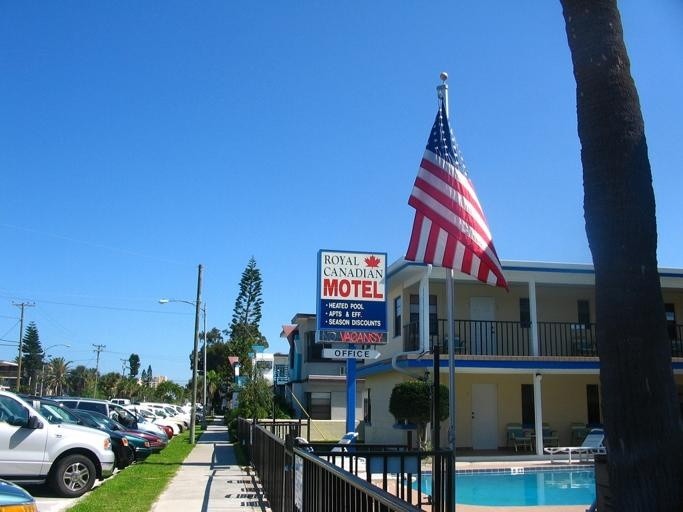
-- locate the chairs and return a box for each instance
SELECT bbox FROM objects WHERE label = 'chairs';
[506,423,607,465]
[444,334,469,354]
[326,431,359,458]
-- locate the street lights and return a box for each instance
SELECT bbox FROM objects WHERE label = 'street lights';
[159,298,207,430]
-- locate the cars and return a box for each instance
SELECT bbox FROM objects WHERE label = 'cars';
[0,388,203,511]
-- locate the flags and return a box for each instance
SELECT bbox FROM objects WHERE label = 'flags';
[403,99,511,295]
